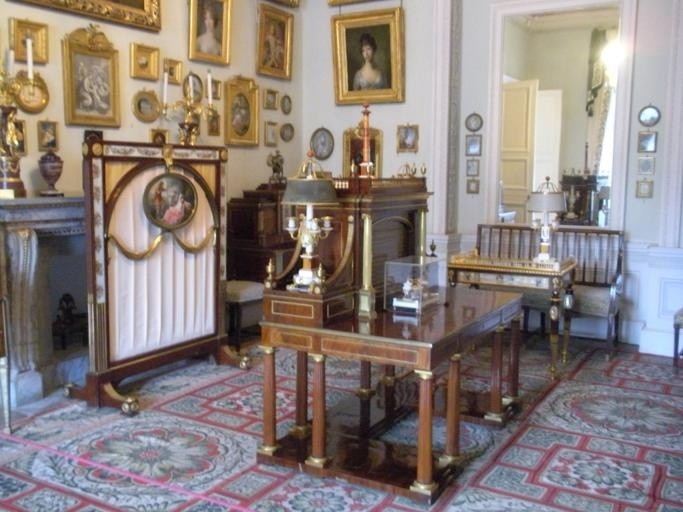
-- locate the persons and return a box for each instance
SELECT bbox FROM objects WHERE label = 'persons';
[352,34,384,91]
[153,181,193,225]
[198,5,223,55]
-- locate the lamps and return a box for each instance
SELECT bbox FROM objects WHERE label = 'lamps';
[525,176,567,264]
[279,151,339,294]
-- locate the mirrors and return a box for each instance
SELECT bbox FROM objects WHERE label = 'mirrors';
[481,1,637,232]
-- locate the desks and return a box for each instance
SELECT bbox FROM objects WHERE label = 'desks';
[257,285,523,505]
[447,248,578,381]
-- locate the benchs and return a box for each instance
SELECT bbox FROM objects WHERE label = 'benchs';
[447,224,625,362]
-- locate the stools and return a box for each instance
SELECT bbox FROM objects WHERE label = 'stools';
[226,279,263,356]
